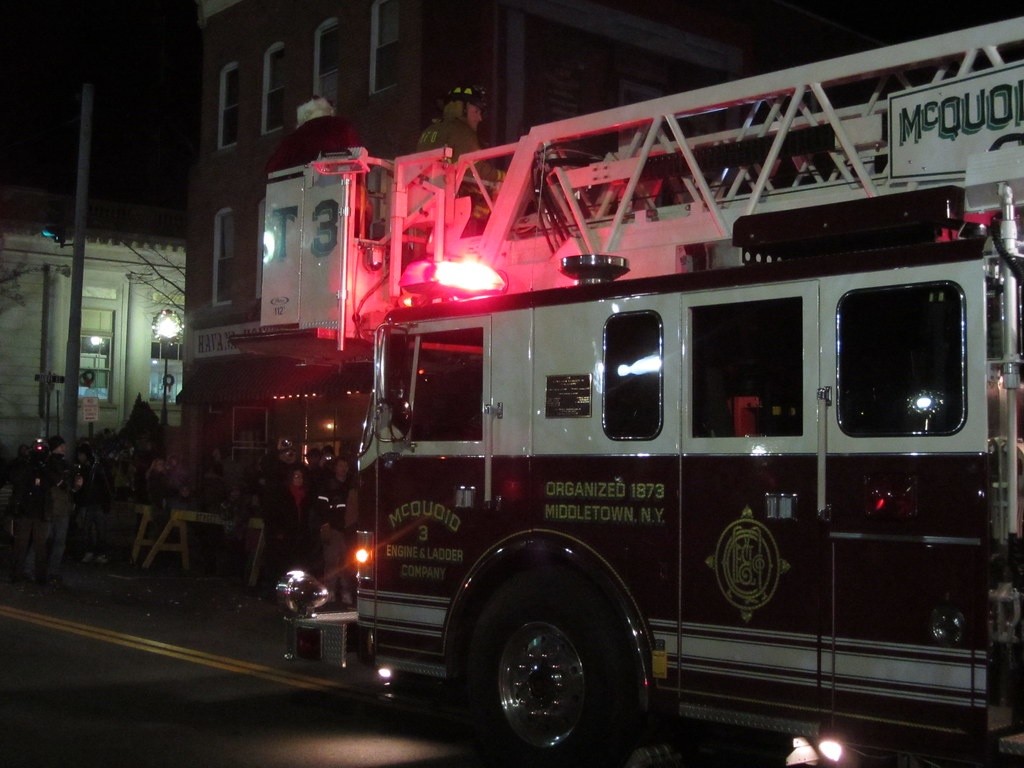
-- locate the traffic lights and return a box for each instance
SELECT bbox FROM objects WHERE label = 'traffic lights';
[41,225,66,249]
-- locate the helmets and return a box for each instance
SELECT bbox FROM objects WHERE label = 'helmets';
[444,87,487,111]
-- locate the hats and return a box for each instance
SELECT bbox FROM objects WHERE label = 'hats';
[49,435,65,452]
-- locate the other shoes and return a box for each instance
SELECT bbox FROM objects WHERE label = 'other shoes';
[48,575,73,594]
[81,553,94,563]
[96,555,108,563]
[21,567,37,583]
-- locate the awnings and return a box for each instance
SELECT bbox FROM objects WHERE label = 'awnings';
[175,357,336,403]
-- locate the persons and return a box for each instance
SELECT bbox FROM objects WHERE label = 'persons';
[205,446,352,546]
[417,82,509,239]
[6,428,155,582]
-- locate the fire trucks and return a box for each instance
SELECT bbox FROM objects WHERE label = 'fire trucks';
[273,184,1024,767]
